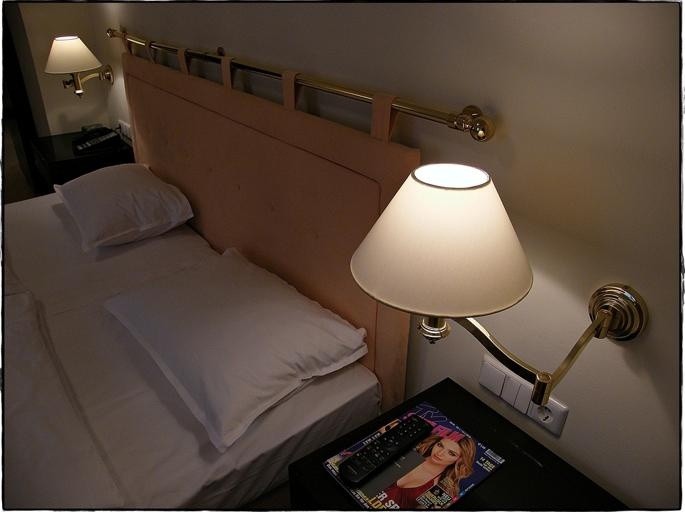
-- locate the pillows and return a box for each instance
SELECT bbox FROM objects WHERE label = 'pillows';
[97,240,371,453]
[50,164,198,255]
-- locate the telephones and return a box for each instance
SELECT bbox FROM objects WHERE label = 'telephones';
[73,127,121,155]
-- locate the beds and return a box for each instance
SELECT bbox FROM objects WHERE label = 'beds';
[3,29,507,510]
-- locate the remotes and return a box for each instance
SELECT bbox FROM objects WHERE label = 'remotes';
[76,131,119,152]
[338,415,433,489]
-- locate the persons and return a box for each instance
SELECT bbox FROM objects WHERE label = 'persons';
[378,432,476,510]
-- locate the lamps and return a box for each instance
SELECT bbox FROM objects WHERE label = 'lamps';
[40,32,117,98]
[346,160,651,408]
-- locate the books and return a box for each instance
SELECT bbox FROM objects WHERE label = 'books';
[324,403,505,511]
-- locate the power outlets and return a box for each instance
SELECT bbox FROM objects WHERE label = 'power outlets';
[525,395,569,438]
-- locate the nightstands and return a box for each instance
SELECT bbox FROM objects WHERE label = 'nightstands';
[15,129,133,191]
[281,371,630,510]
